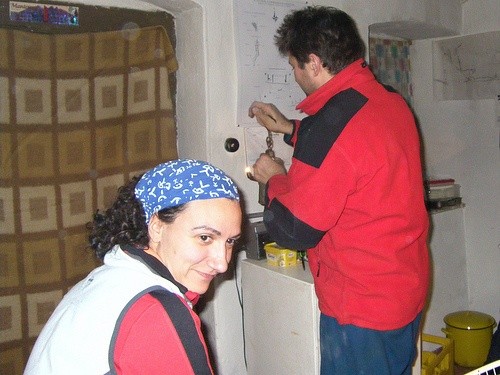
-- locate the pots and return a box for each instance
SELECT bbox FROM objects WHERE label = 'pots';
[441,310,496,367]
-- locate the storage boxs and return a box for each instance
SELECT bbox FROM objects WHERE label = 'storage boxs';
[421,333,454,375]
[264,242,297,268]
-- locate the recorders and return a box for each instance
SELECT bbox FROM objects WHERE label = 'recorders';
[242,212,277,260]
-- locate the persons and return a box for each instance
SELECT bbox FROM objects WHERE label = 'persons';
[248,5,431,375]
[23,158,243,375]
